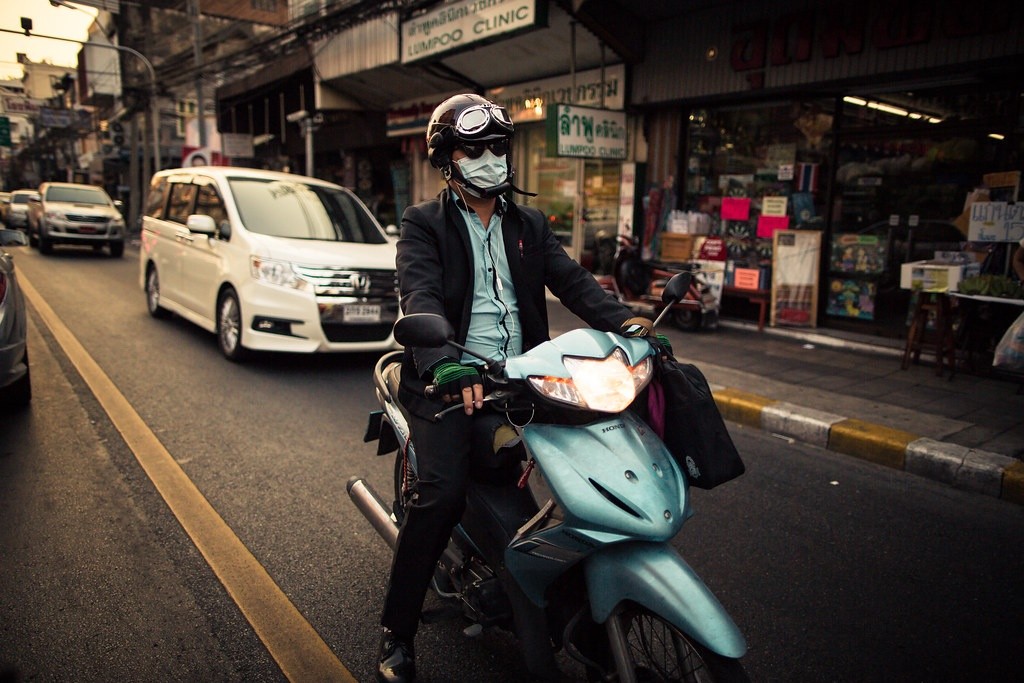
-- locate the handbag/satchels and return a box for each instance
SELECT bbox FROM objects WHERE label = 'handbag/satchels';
[646,337,745,490]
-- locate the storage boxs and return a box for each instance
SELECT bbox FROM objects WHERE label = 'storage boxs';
[900,259,966,292]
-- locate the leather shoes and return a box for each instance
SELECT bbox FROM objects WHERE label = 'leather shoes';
[375,628,416,683]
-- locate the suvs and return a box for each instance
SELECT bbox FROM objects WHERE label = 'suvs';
[137,164,411,366]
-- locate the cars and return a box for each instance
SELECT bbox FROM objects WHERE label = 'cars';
[0,246,33,408]
[0,189,36,231]
[25,181,127,258]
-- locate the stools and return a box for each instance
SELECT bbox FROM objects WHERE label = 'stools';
[900,292,958,375]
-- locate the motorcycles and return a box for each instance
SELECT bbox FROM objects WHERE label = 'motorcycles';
[342,268,752,683]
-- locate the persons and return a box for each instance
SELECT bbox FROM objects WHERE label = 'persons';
[374,93,655,683]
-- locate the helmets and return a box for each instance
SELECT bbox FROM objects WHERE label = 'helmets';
[426,94,517,169]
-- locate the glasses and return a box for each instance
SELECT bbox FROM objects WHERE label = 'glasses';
[454,141,511,159]
[453,102,514,135]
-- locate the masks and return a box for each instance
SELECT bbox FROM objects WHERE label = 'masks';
[452,149,507,198]
